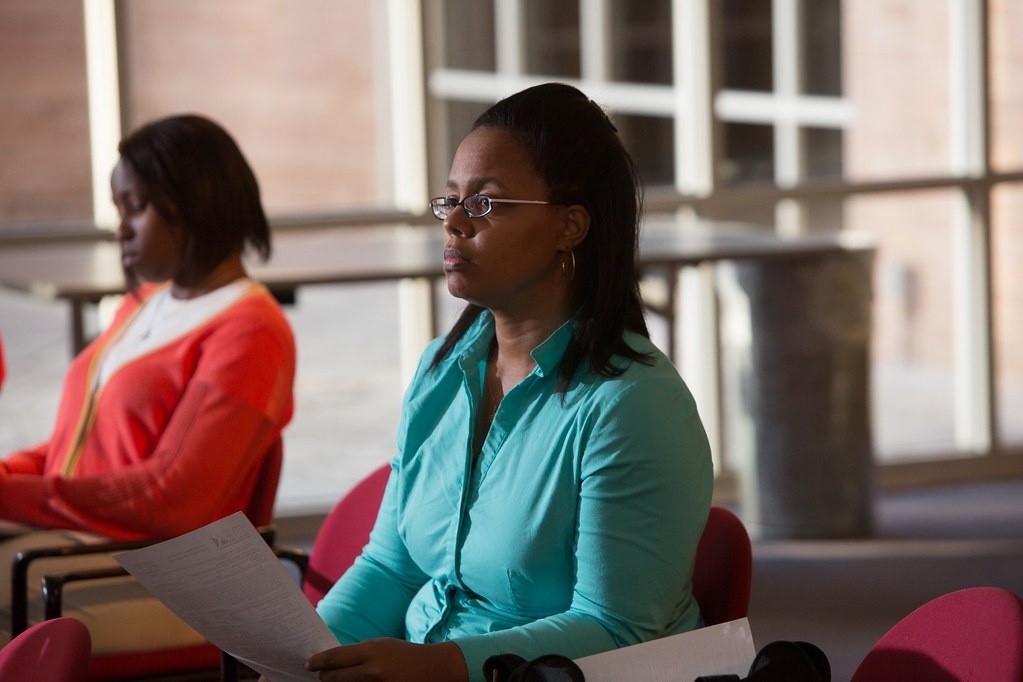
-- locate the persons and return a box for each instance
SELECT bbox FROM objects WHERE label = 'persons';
[0,113,296,539]
[260,82,714,682]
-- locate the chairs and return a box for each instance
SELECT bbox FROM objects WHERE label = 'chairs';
[0,437,391,682]
[686,508,1022,682]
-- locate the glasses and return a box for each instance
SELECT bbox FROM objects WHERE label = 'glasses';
[428,194,566,220]
